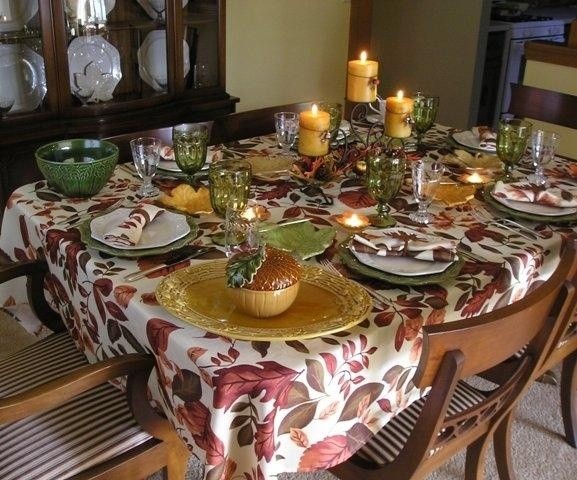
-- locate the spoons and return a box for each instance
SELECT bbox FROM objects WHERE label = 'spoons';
[211,215,313,247]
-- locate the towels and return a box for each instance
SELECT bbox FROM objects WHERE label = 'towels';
[350,227,458,266]
[490,178,576,211]
[147,143,179,161]
[104,202,164,248]
[470,124,496,153]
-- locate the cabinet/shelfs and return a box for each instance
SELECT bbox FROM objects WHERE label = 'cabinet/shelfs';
[1,0,240,277]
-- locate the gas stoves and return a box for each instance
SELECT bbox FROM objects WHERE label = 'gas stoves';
[489,13,565,40]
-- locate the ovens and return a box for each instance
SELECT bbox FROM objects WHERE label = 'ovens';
[498,35,565,121]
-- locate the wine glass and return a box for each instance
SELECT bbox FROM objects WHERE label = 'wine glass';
[496,117,531,179]
[406,159,449,224]
[363,147,405,228]
[128,136,163,199]
[526,128,561,182]
[312,100,341,149]
[171,123,211,186]
[274,111,300,157]
[410,94,439,149]
[205,158,253,245]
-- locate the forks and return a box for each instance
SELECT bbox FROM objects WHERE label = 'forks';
[319,258,394,307]
[466,204,546,243]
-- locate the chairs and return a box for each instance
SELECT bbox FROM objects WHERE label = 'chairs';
[476,253,577,479]
[96,119,214,164]
[0,257,189,480]
[502,80,575,164]
[328,244,577,479]
[214,101,323,143]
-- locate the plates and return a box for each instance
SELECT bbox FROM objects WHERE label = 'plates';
[250,216,336,263]
[481,182,576,224]
[337,232,466,286]
[153,164,217,177]
[446,127,495,157]
[244,156,296,182]
[427,182,475,209]
[459,157,504,176]
[326,118,356,148]
[154,259,374,342]
[79,206,196,259]
[0,1,191,114]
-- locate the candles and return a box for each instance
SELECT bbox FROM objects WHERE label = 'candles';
[383,90,413,138]
[345,49,380,103]
[296,105,328,156]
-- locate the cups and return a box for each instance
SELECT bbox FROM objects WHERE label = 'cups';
[297,111,330,157]
[222,197,260,259]
[345,60,378,103]
[383,97,413,139]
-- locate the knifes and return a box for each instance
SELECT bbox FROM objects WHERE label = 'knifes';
[122,245,214,285]
[120,164,158,187]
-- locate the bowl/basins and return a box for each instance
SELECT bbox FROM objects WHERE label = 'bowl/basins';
[33,138,119,199]
[505,2,529,13]
[222,273,301,320]
